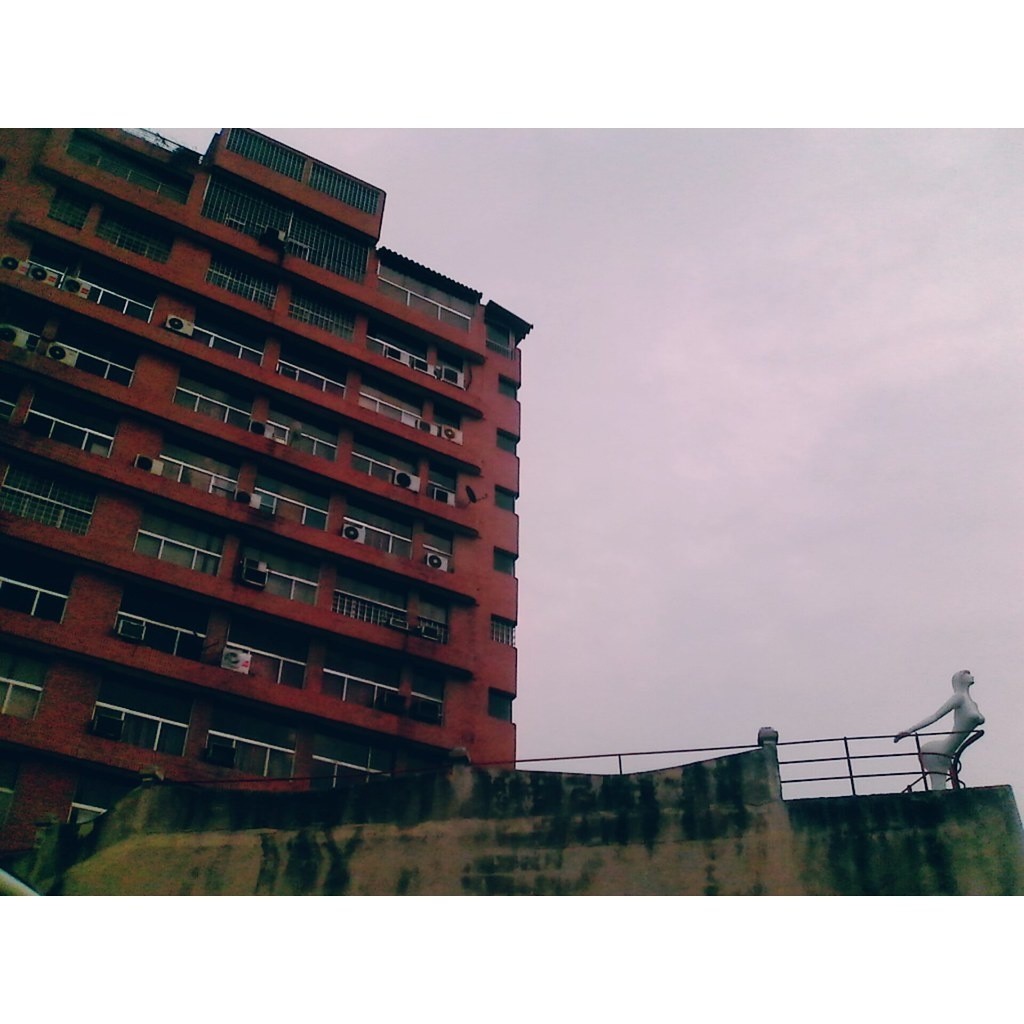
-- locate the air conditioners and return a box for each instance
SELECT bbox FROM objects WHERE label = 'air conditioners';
[134,454,164,476]
[236,557,269,588]
[208,743,237,767]
[432,487,456,507]
[265,225,286,243]
[248,417,276,438]
[61,276,92,299]
[393,469,422,493]
[342,524,366,545]
[440,366,465,390]
[413,359,436,376]
[220,647,252,674]
[421,625,440,642]
[166,314,194,337]
[1,255,30,276]
[0,324,30,348]
[233,487,261,509]
[117,619,147,640]
[440,426,464,444]
[385,347,410,366]
[386,617,409,631]
[417,419,438,436]
[425,554,448,571]
[45,342,79,368]
[94,715,124,739]
[29,265,58,285]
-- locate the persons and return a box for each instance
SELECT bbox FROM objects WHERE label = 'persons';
[894,670,985,790]
